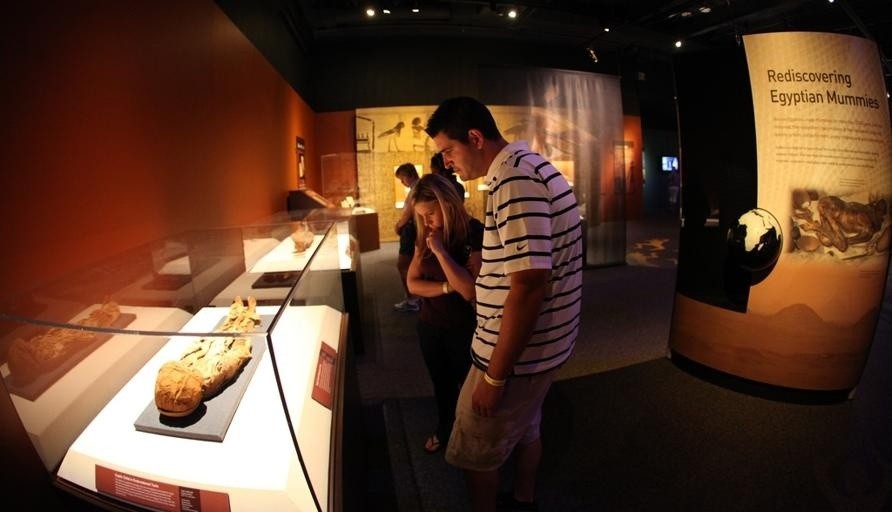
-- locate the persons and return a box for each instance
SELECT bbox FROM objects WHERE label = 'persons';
[394,162,422,312]
[406,172,483,454]
[423,95,584,512]
[430,153,465,203]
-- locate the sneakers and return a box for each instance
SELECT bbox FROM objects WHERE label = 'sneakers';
[495,491,539,512]
[393,296,425,312]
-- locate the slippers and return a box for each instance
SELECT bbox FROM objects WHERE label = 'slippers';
[423,433,441,453]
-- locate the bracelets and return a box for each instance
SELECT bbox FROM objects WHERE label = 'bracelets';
[441,281,450,295]
[485,371,506,387]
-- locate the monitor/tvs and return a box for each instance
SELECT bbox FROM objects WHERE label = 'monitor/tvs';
[660,155,679,171]
